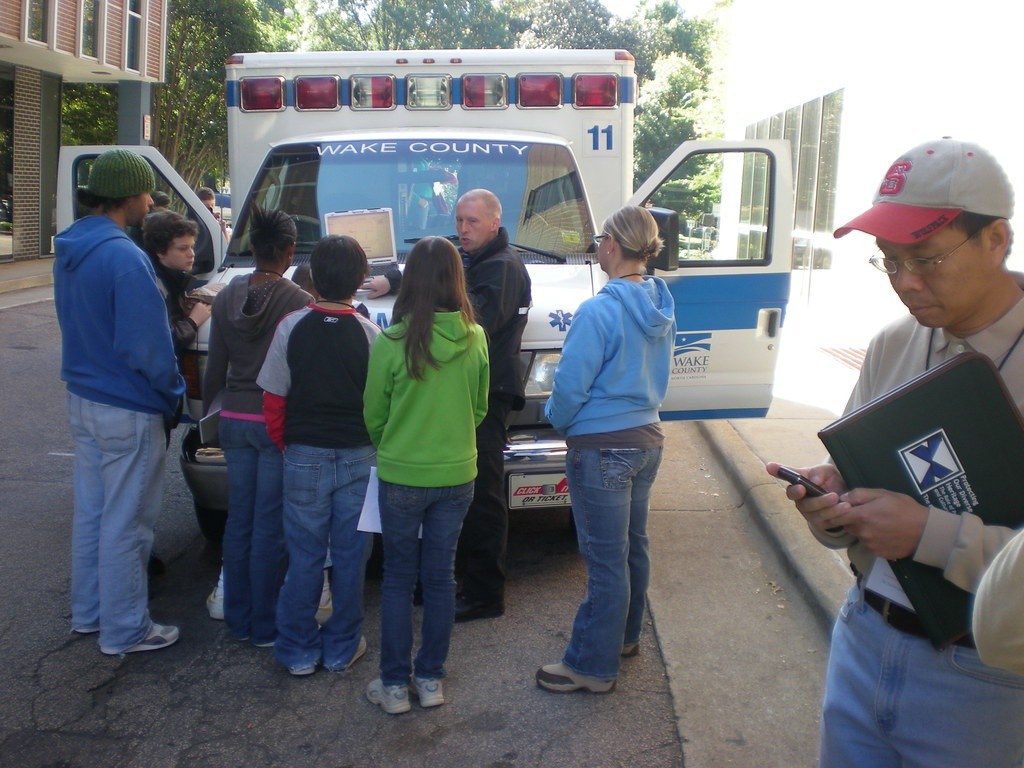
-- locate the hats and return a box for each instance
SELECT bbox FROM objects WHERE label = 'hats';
[834,136,1015,244]
[89,149,155,198]
[196,187,215,201]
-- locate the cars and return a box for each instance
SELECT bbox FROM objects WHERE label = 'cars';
[215,193,231,228]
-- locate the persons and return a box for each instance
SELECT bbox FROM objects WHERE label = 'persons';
[186,186,230,277]
[52,149,187,654]
[364,238,490,714]
[141,208,213,578]
[357,188,532,622]
[201,211,322,648]
[767,136,1024,768]
[256,234,383,675]
[130,191,171,249]
[536,205,677,695]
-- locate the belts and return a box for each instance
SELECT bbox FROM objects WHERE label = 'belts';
[864,589,976,648]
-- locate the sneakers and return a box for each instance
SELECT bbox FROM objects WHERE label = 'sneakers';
[411,672,444,708]
[207,586,225,619]
[536,661,617,694]
[101,623,179,654]
[366,678,411,714]
[621,641,638,657]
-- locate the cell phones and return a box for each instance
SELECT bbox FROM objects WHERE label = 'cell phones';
[778,466,841,504]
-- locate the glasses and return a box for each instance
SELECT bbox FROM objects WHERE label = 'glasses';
[869,229,982,275]
[593,234,607,245]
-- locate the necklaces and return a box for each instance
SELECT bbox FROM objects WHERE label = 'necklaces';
[315,300,356,309]
[618,272,644,278]
[253,269,283,278]
[925,327,1024,371]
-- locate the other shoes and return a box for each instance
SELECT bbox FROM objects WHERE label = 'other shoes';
[348,635,367,668]
[149,556,165,574]
[455,593,505,621]
[289,665,315,675]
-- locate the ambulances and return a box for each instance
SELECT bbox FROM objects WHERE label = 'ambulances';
[56,49,793,539]
